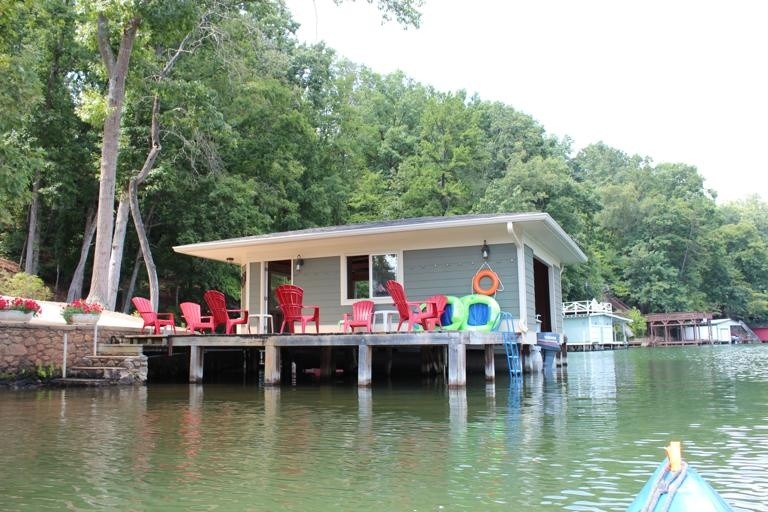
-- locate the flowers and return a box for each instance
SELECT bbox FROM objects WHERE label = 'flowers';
[0,296,42,317]
[61,297,104,325]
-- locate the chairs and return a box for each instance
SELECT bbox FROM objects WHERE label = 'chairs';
[342,300,375,334]
[276,284,320,334]
[387,279,447,333]
[131,290,247,335]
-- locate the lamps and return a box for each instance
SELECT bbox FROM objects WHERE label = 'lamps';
[295,254,305,271]
[481,239,490,259]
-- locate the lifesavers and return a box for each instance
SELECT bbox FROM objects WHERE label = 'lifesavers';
[473,270,499,296]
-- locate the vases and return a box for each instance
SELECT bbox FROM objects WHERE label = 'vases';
[0,309,33,321]
[71,313,101,325]
